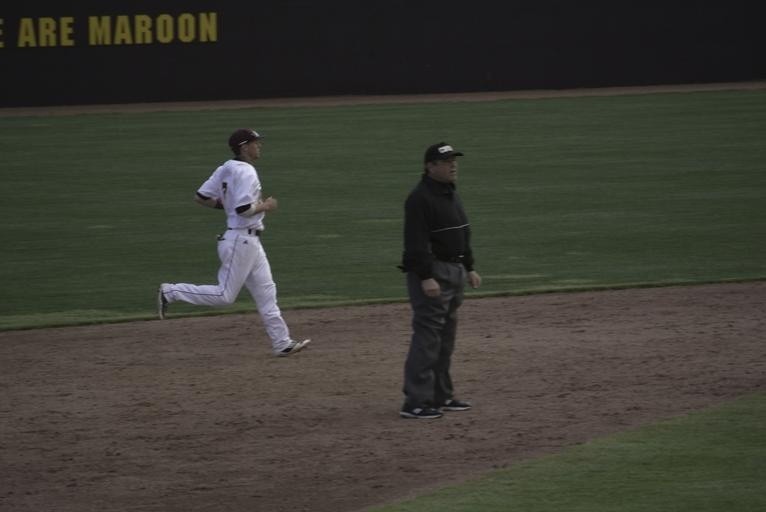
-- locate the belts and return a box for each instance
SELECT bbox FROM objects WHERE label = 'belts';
[228,226,259,236]
[431,253,466,264]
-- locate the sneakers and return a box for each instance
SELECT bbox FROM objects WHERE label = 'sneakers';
[437,396,473,411]
[275,339,310,357]
[400,401,444,419]
[158,282,170,320]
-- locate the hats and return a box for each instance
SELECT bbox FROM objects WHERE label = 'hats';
[424,142,464,161]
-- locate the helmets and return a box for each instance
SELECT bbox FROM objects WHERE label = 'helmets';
[229,128,265,149]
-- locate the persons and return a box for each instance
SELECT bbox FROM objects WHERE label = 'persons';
[156,128,313,357]
[396,142,482,420]
